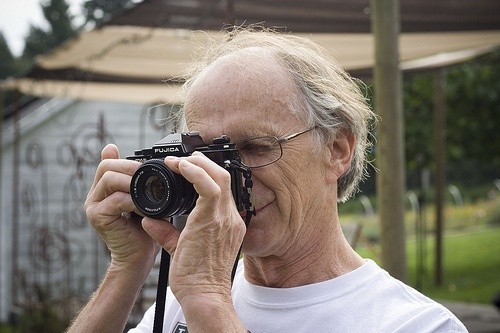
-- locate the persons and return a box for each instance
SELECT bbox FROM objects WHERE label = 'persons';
[61,23,471,333]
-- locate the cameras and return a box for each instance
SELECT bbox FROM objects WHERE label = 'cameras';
[125,128,244,223]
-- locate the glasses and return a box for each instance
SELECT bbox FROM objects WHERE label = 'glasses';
[235,124,318,168]
